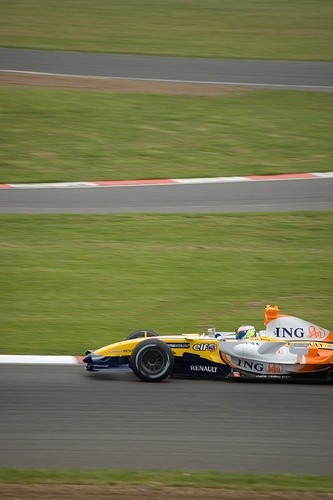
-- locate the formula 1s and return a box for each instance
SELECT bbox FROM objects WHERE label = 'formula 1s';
[79,303,333,382]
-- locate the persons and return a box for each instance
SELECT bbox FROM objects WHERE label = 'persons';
[236,324,255,340]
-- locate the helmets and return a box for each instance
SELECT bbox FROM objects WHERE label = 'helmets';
[235,324,257,339]
